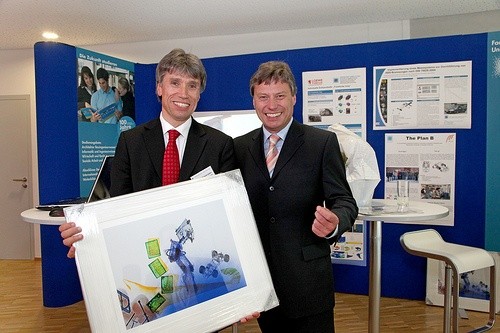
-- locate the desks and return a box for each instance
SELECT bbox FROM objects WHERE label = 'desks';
[20,197,88,227]
[355,198,450,333]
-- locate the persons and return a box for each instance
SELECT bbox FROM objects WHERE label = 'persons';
[232,60,359,333]
[58,49,261,333]
[77,65,97,121]
[89,67,123,124]
[112,78,135,123]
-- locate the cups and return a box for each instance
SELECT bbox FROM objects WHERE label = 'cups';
[397,181,409,213]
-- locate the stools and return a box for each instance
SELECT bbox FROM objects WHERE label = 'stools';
[400,230,497,333]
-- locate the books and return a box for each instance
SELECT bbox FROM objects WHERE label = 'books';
[81,102,117,123]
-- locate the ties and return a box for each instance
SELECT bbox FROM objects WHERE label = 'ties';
[264,134,281,178]
[162,129,182,187]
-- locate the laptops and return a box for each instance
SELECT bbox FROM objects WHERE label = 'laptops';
[35,155,114,210]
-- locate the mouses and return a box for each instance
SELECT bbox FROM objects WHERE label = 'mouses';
[49,208,65,217]
